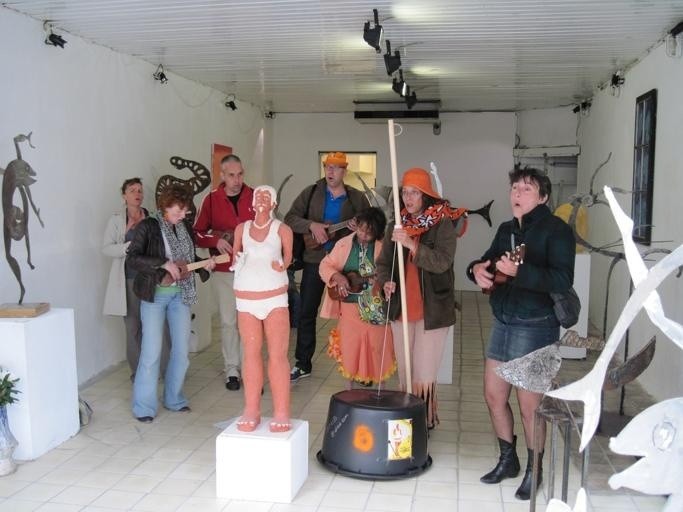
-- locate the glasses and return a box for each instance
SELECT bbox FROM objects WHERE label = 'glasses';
[324,165,345,170]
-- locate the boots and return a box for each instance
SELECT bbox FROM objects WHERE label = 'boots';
[478,436,520,484]
[514,448,545,500]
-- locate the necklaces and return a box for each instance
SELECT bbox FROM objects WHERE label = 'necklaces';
[253,219,272,230]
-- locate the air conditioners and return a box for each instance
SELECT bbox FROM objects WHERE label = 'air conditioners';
[353,100,441,125]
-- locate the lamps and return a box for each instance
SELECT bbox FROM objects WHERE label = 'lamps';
[383,51,402,76]
[404,96,418,109]
[49,32,68,49]
[392,78,408,97]
[158,72,168,85]
[363,22,385,52]
[224,102,239,112]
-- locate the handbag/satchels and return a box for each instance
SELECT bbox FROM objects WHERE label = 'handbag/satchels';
[548,284,583,330]
[285,184,317,270]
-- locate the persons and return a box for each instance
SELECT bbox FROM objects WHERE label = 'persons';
[375,166,457,430]
[281,150,371,382]
[194,153,264,396]
[228,184,295,434]
[318,207,398,389]
[101,177,157,383]
[127,184,216,424]
[465,168,576,501]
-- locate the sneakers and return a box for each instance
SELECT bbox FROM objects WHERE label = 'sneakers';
[288,366,311,381]
[224,375,241,390]
[180,407,191,413]
[136,414,152,423]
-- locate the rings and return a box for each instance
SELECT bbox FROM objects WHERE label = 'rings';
[340,287,344,290]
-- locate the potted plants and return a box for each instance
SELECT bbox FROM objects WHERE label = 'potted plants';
[0,365,22,477]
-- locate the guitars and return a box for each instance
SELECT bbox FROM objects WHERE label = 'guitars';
[303,217,355,250]
[329,270,376,300]
[481,243,525,294]
[160,254,230,287]
[211,230,234,251]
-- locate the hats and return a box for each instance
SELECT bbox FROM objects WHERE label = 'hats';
[401,167,441,201]
[322,151,349,166]
